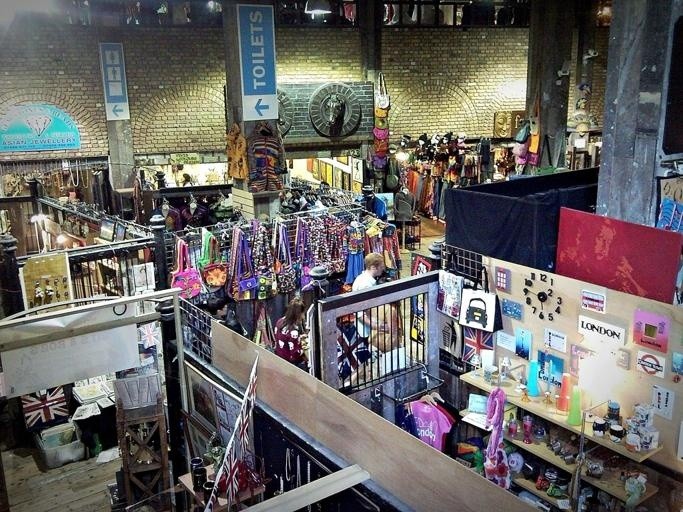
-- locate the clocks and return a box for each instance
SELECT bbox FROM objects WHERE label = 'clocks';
[522,273,561,321]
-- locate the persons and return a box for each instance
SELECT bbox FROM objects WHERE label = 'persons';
[274,299,309,371]
[181,174,193,187]
[167,165,178,185]
[349,252,389,338]
[369,301,401,353]
[207,293,229,326]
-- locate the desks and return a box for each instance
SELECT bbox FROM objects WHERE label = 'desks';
[178,462,264,511]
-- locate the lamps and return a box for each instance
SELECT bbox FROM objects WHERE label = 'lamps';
[305,1,331,14]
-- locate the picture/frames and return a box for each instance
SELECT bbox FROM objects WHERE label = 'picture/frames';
[341,172,350,190]
[311,159,319,181]
[351,157,364,183]
[99,219,116,241]
[307,159,313,173]
[115,223,126,242]
[351,180,362,194]
[325,162,333,188]
[320,161,326,183]
[337,158,346,163]
[334,168,342,189]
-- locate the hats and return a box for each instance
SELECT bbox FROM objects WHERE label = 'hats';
[362,185,374,191]
[444,131,453,139]
[419,133,427,142]
[309,266,329,277]
[388,143,398,150]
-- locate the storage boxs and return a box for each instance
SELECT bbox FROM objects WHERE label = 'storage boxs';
[34,420,84,469]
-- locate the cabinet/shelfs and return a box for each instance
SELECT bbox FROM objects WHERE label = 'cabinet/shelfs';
[116,397,172,512]
[459,367,665,511]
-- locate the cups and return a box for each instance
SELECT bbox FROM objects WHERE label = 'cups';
[202,482,217,506]
[592,420,608,437]
[193,469,206,492]
[625,435,641,452]
[191,458,203,473]
[610,425,626,443]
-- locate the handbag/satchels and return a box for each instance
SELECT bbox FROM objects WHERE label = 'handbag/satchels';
[374,116,389,129]
[171,235,201,299]
[513,121,530,142]
[272,221,297,294]
[374,106,388,117]
[386,161,399,189]
[527,149,539,166]
[456,265,503,334]
[199,226,227,289]
[377,72,390,109]
[254,225,279,300]
[529,77,541,136]
[514,154,529,164]
[294,215,316,292]
[511,136,529,158]
[527,96,540,154]
[373,127,388,139]
[373,136,388,152]
[229,225,256,301]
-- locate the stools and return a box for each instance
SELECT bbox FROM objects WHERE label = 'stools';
[407,217,421,250]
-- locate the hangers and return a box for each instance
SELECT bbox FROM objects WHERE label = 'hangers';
[419,390,430,404]
[426,390,437,406]
[404,397,413,415]
[430,386,445,403]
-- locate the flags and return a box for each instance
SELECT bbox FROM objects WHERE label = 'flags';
[334,326,371,378]
[237,407,251,453]
[140,323,159,349]
[248,372,259,405]
[223,440,241,504]
[461,326,492,367]
[19,385,71,429]
[206,486,218,512]
